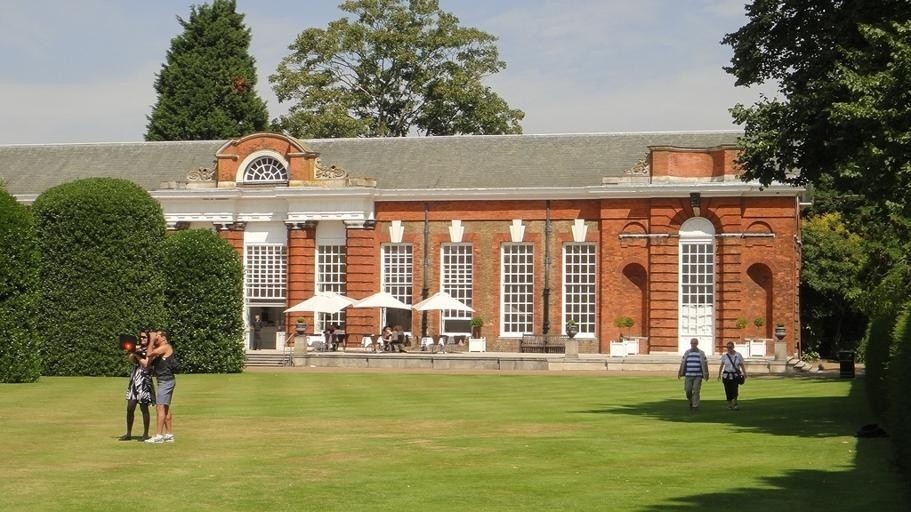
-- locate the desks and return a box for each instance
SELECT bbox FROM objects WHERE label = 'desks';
[306,336,325,345]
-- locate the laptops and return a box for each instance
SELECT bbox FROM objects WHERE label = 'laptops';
[119,333,144,355]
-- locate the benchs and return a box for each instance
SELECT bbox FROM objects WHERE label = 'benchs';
[519,333,570,353]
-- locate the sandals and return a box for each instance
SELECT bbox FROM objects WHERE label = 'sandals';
[730,403,738,410]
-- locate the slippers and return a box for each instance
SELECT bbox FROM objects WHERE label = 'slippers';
[119,435,132,441]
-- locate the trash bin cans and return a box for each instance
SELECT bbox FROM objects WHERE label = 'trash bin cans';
[839,351,855,379]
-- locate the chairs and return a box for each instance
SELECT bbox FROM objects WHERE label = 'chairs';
[331,334,466,356]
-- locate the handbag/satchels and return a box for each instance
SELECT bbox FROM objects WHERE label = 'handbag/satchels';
[737,374,744,384]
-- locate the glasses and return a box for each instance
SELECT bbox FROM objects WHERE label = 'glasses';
[140,335,148,338]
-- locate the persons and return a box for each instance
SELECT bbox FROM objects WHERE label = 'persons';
[252,311,266,350]
[718,341,749,411]
[144,328,176,443]
[320,322,404,354]
[678,338,710,411]
[118,329,157,442]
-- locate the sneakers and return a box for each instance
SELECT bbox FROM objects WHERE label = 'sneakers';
[144,434,174,444]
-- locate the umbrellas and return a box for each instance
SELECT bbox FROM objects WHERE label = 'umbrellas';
[352,287,412,313]
[280,289,358,316]
[411,291,477,311]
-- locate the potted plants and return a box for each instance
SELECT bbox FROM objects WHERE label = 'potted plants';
[775,318,787,333]
[468,317,483,338]
[295,317,306,328]
[609,316,639,357]
[568,320,577,331]
[732,317,766,359]
